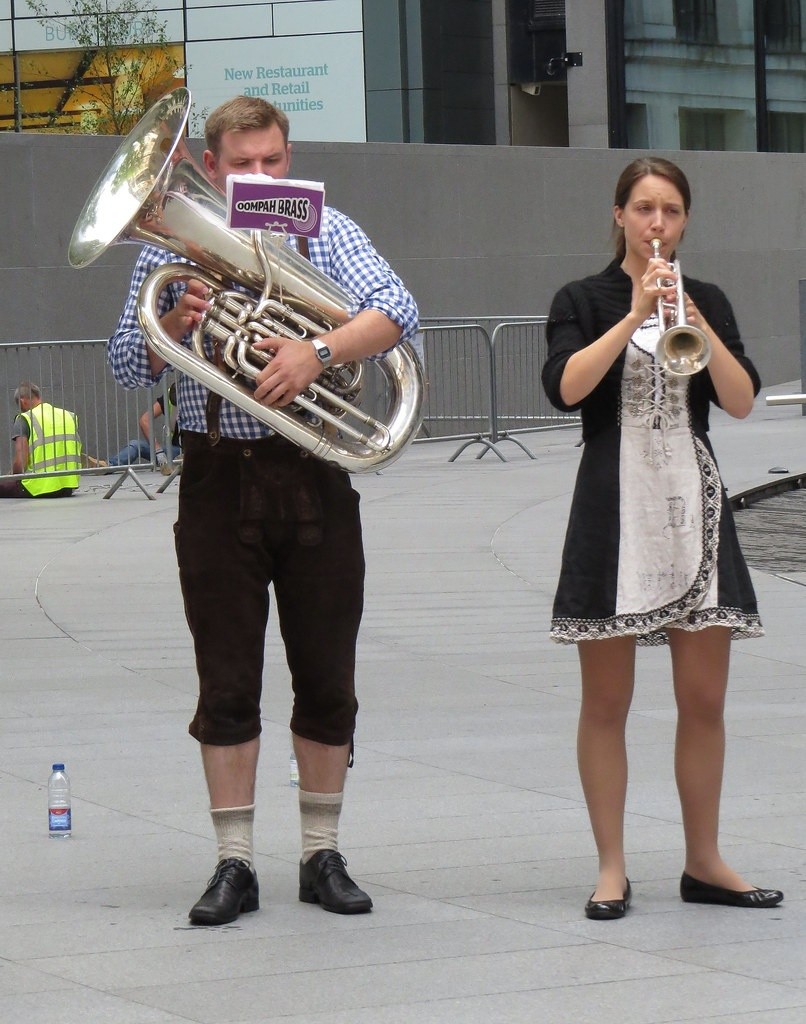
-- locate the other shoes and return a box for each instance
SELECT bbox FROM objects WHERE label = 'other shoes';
[583,878,632,921]
[680,871,784,909]
[160,463,180,476]
[80,453,112,475]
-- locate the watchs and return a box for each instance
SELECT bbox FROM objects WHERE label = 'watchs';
[311,336,332,370]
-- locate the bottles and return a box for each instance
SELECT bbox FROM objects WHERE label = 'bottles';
[47,763,71,838]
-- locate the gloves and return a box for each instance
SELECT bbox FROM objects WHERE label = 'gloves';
[155,449,167,466]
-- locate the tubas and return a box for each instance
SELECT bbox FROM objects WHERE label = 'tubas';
[67,88,430,474]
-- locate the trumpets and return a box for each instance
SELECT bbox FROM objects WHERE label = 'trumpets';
[649,238,713,376]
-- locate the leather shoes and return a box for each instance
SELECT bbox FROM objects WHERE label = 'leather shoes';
[297,848,374,915]
[187,857,260,924]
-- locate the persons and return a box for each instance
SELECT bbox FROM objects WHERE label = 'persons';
[106,96,422,924]
[541,154,784,919]
[0,380,83,498]
[81,382,182,477]
